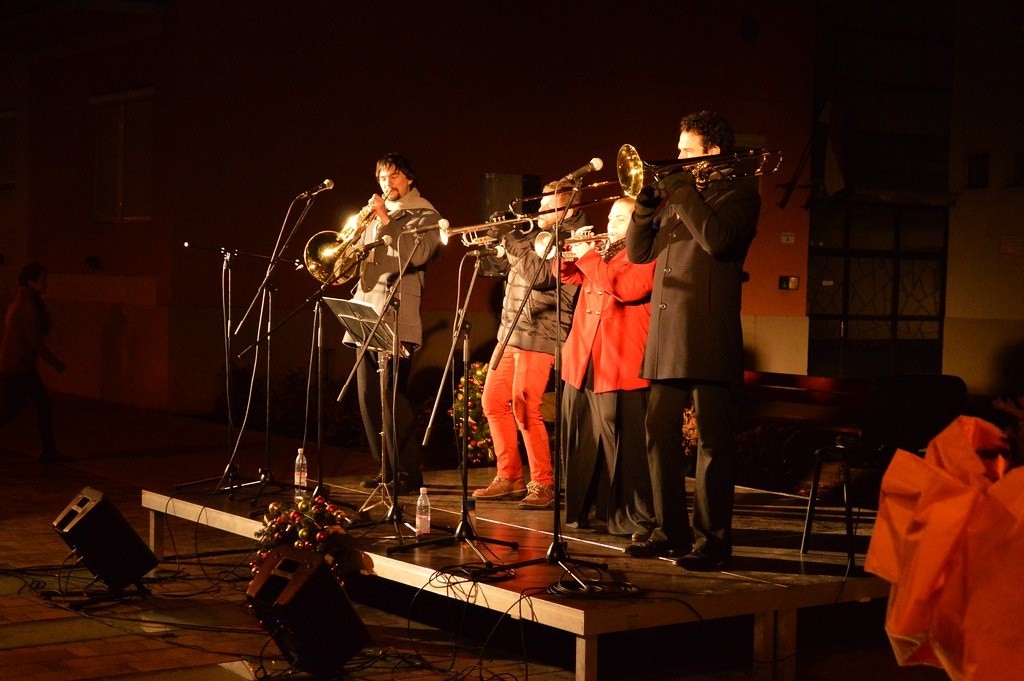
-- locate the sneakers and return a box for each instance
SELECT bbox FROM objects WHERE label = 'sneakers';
[472,476,527,498]
[519,481,555,507]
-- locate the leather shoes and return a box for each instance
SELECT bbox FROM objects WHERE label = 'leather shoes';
[360,472,389,488]
[627,528,693,557]
[678,538,733,570]
[378,480,423,495]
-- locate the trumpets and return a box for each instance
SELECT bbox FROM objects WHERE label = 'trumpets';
[533,231,612,263]
[437,215,539,246]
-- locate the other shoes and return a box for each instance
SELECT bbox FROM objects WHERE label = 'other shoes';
[565,522,585,528]
[631,527,650,541]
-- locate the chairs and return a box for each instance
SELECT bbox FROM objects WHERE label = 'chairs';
[743,373,936,576]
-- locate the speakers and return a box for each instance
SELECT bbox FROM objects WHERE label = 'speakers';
[52,486,161,593]
[245,544,370,675]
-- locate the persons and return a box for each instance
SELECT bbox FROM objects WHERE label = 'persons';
[552,197,658,541]
[341,153,443,496]
[471,179,590,508]
[623,110,761,569]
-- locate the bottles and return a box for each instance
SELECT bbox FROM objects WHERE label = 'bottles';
[295,448,307,498]
[460,498,476,545]
[415,488,431,537]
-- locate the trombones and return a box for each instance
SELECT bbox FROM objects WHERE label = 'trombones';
[507,144,784,220]
[303,188,393,287]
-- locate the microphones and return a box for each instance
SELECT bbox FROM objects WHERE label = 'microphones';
[296,178,334,200]
[466,246,505,258]
[354,234,393,253]
[402,218,449,235]
[559,157,603,183]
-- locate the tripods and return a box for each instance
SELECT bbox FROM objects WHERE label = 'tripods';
[320,297,411,519]
[385,258,520,569]
[171,197,371,519]
[469,184,608,594]
[342,236,457,546]
